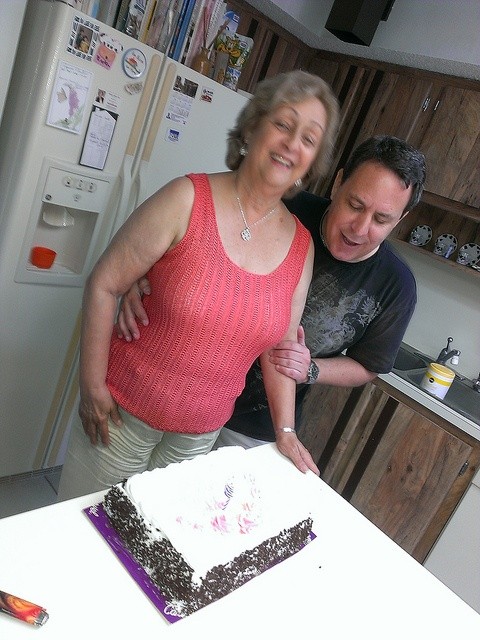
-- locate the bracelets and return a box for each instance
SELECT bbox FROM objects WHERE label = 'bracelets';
[275,426,297,437]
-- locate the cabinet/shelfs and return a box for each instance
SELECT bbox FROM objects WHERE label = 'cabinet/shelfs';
[388,198,480,282]
[296,353,480,568]
[224,0,311,96]
[296,49,379,201]
[344,59,480,225]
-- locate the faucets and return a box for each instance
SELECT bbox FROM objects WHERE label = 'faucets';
[433,336,461,366]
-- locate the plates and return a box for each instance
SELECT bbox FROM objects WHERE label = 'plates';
[409,224,433,246]
[457,240,479,266]
[433,233,459,257]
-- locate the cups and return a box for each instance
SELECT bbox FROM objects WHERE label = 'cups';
[29,244,57,270]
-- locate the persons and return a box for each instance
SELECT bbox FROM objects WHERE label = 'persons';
[115,135,431,452]
[55,70,341,506]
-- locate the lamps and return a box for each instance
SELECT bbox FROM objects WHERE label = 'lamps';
[323,1,396,51]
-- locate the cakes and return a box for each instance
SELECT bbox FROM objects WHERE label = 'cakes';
[90,445,314,615]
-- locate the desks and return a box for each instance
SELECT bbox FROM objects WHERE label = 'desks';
[1,441,480,632]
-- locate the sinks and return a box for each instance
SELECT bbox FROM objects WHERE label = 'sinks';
[390,342,427,370]
[407,372,479,426]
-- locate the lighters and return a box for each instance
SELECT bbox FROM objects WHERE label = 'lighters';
[0,591,49,627]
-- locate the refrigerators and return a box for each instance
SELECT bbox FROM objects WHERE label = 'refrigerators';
[0,1,269,480]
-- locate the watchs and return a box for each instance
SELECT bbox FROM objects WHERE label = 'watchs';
[304,359,320,385]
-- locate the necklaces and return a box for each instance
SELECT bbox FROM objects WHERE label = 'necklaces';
[233,168,281,241]
